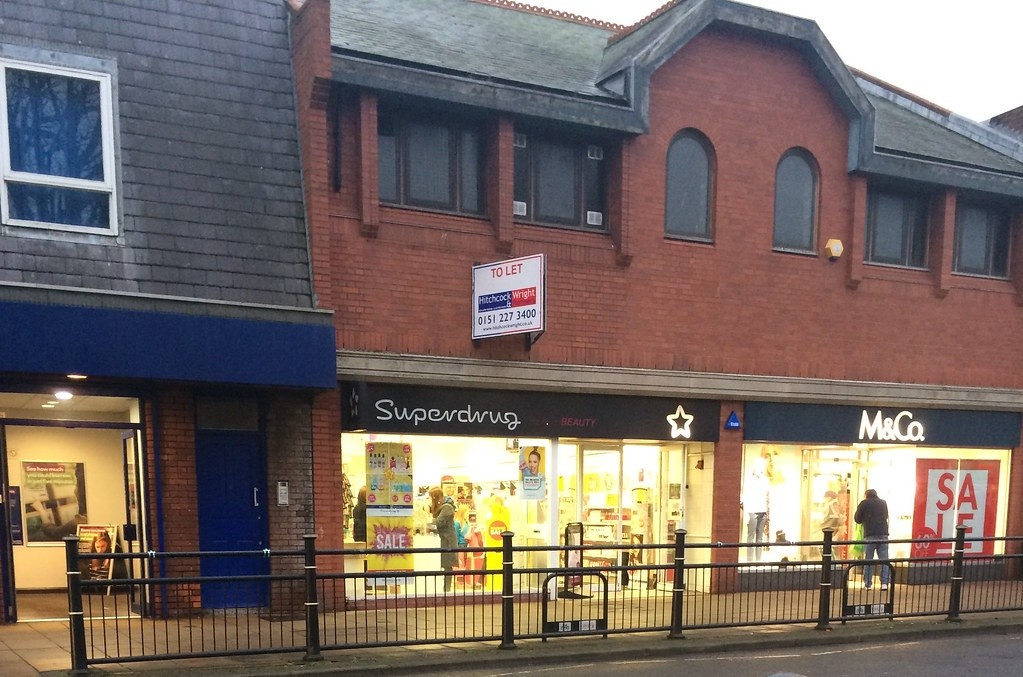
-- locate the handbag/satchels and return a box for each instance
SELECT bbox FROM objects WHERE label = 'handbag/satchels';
[850,524,864,557]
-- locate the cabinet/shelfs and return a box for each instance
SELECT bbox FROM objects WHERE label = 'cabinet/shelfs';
[582,507,632,584]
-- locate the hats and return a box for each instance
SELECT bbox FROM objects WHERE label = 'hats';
[823,490,838,498]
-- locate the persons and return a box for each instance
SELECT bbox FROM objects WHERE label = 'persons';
[854,489,889,589]
[455,508,471,560]
[89,529,112,568]
[353,485,372,589]
[528,451,545,481]
[413,486,459,591]
[821,490,840,560]
[30,463,87,541]
[742,458,767,563]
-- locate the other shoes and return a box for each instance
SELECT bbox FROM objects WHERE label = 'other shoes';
[881,584,887,590]
[863,586,871,591]
